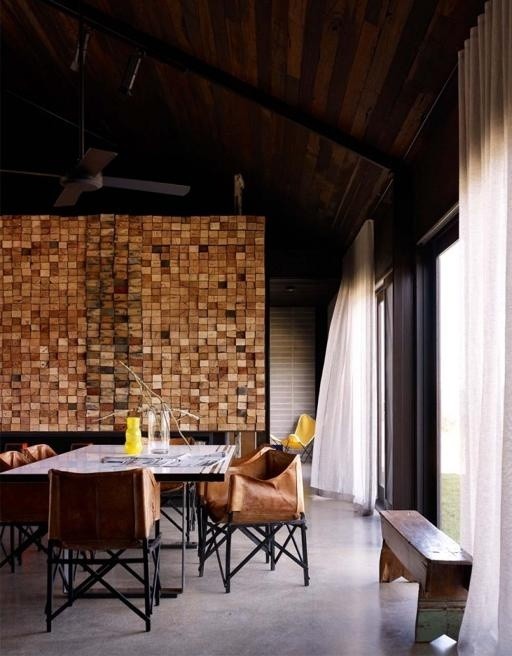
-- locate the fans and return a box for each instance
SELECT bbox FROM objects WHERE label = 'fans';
[0,20,190,208]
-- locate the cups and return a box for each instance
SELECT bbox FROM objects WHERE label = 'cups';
[148,409,171,454]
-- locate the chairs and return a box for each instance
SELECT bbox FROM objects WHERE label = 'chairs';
[272,412,318,464]
[0,444,310,634]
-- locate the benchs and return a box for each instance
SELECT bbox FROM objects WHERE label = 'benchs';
[376,509,474,645]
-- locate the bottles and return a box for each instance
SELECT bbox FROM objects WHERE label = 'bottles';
[124,417,142,455]
